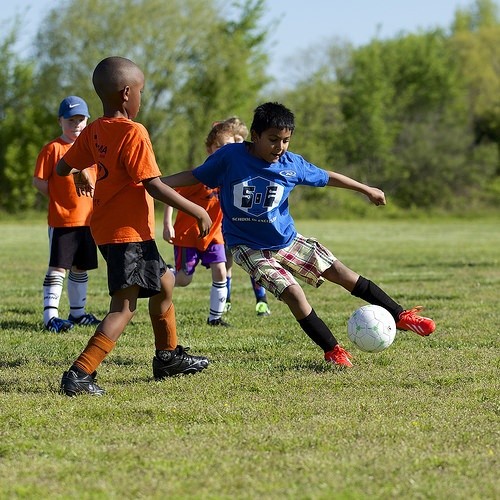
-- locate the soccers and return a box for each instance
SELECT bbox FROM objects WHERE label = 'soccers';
[347,304,396,353]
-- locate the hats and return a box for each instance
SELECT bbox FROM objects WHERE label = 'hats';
[58,95,91,119]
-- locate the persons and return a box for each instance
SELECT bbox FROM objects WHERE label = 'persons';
[33,95,102,335]
[163,116,271,328]
[56,56,212,399]
[159,102,435,368]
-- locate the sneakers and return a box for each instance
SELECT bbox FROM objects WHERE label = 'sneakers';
[166,264,173,269]
[152,344,210,380]
[68,312,102,325]
[223,301,231,313]
[255,301,271,317]
[396,305,436,337]
[207,317,229,326]
[60,370,108,396]
[45,316,74,333]
[324,344,353,368]
